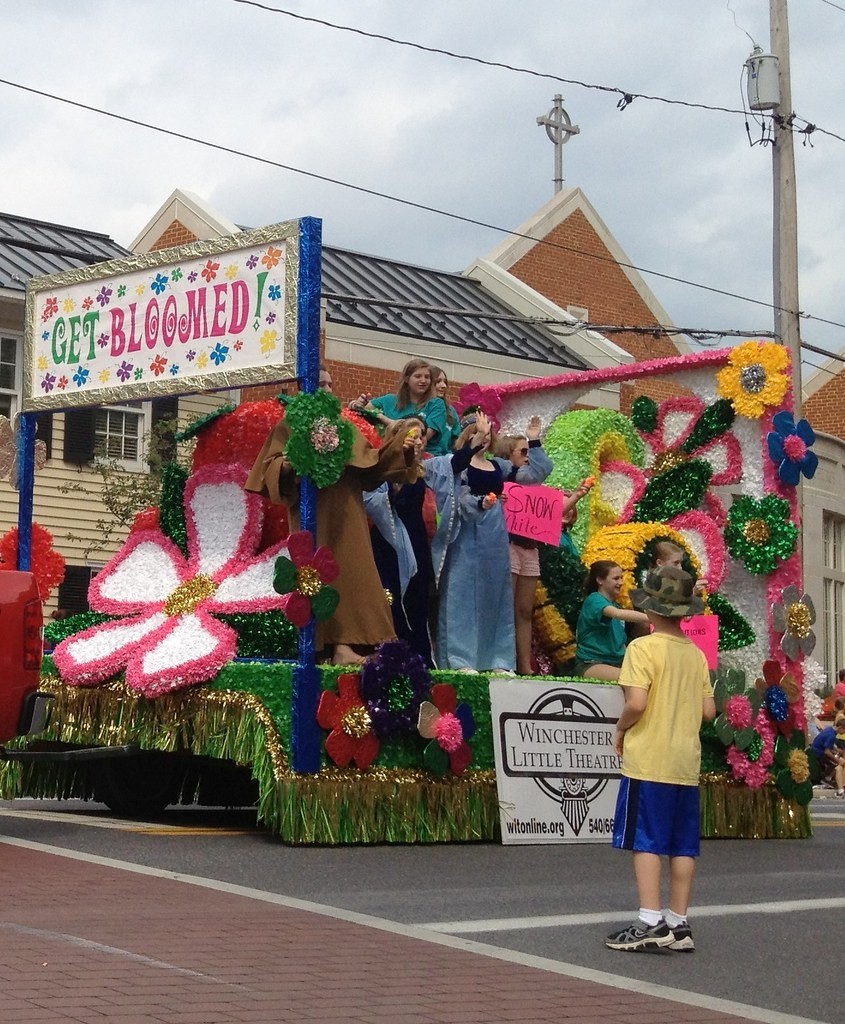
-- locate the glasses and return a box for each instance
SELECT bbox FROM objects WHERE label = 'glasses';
[513,447,529,457]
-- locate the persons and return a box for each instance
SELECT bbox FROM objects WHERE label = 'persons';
[492,431,594,675]
[812,718,845,798]
[573,560,656,681]
[832,697,845,788]
[653,525,708,622]
[425,364,463,454]
[434,409,553,676]
[835,671,845,696]
[541,487,591,620]
[360,356,449,454]
[609,567,718,949]
[364,411,491,670]
[242,364,422,670]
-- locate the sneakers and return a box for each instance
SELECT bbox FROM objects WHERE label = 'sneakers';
[659,920,695,951]
[603,915,676,953]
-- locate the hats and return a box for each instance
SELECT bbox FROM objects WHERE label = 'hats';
[627,566,706,618]
